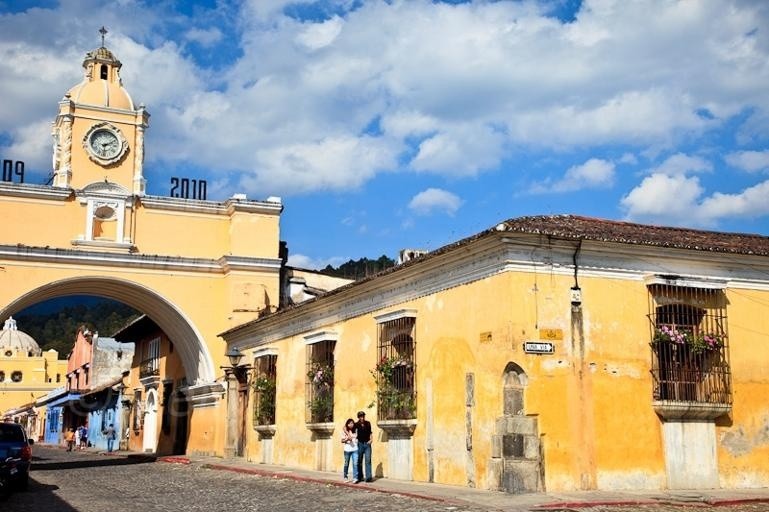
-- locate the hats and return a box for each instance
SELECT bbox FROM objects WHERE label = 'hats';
[358,412,365,417]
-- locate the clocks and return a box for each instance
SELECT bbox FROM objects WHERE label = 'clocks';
[82,121,129,166]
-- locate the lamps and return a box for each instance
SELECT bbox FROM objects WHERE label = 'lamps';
[224,346,256,369]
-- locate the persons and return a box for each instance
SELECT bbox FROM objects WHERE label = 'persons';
[342,411,373,482]
[124,423,130,450]
[65,419,88,454]
[340,418,360,484]
[104,423,116,452]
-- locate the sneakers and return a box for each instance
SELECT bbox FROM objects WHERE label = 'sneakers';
[343,478,359,484]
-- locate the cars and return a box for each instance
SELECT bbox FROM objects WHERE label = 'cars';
[0,423,34,490]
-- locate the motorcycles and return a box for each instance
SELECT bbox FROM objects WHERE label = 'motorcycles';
[0,456,29,497]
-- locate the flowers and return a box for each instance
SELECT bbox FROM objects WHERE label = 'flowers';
[307,363,333,422]
[650,326,723,354]
[367,354,416,419]
